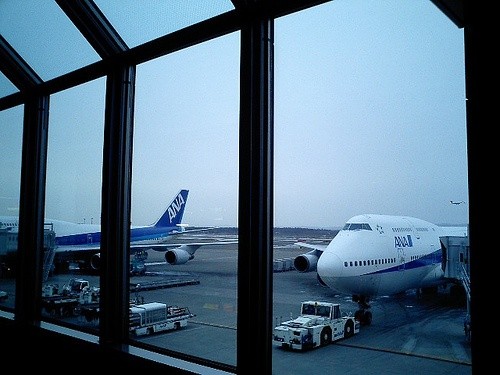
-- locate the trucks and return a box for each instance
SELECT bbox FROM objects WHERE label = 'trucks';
[40,278,100,318]
[272,301,359,351]
[128,301,194,338]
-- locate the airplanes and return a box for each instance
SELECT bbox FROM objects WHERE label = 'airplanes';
[293,213,470,342]
[449,200,468,206]
[0,188,238,275]
